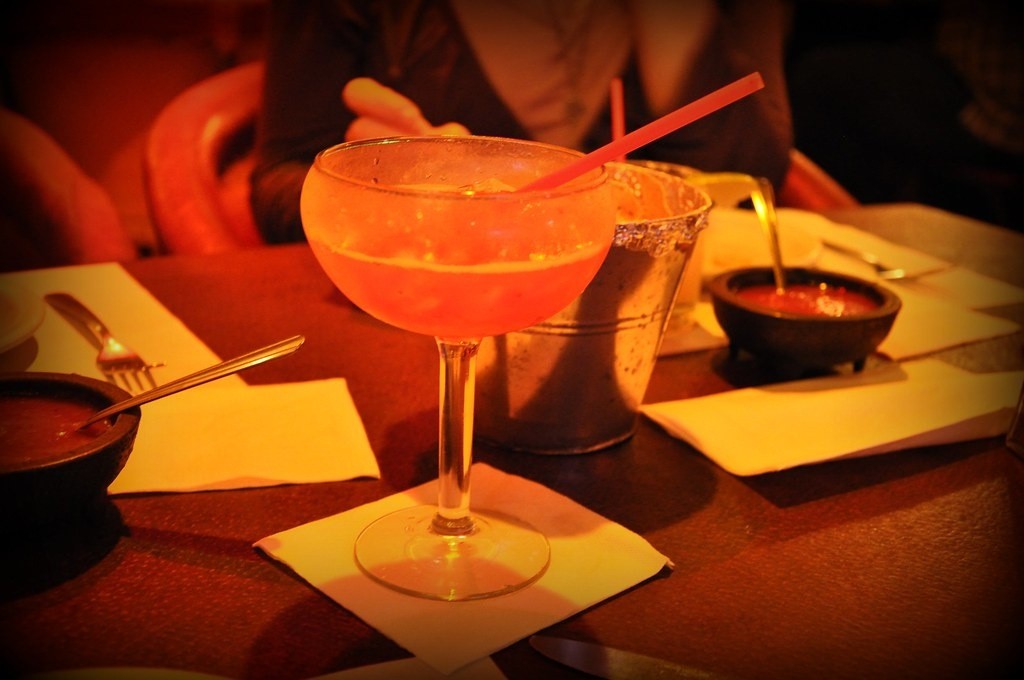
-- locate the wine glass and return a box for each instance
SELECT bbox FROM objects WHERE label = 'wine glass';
[299,134,617,598]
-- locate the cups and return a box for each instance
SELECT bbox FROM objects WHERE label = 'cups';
[480,160,715,455]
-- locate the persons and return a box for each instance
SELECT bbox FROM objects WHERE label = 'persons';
[250,0,790,248]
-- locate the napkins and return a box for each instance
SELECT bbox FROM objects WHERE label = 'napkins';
[640,357,1023,477]
[252,463,675,677]
[107,377,381,496]
[1,262,249,395]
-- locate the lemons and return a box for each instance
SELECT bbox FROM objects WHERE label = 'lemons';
[680,173,756,208]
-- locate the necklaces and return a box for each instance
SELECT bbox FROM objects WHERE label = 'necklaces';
[543,9,618,119]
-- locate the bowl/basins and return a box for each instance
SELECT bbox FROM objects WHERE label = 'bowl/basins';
[0,368,143,553]
[706,266,904,373]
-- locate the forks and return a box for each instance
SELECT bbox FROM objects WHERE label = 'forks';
[44,292,166,374]
[826,242,944,283]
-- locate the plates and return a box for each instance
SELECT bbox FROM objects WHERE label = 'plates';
[0,277,46,354]
[678,198,824,275]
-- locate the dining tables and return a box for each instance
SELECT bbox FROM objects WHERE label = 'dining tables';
[0,203,1024,679]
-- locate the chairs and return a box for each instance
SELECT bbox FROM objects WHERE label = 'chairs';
[145,57,862,258]
